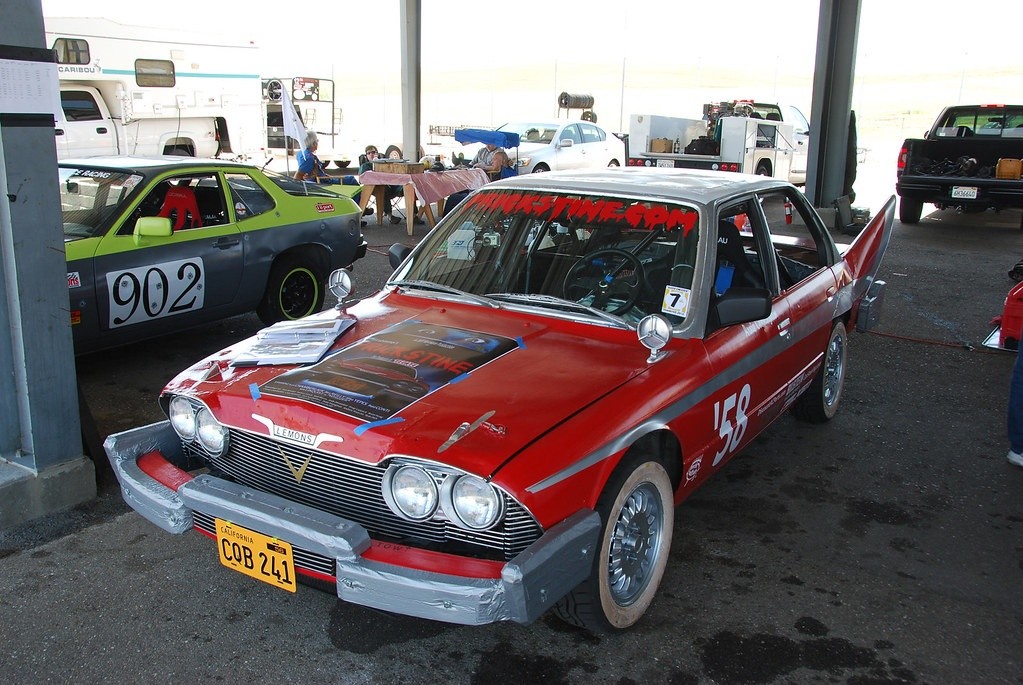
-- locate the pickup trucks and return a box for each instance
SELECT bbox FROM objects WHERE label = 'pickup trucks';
[54,83,220,161]
[895,104,1022,228]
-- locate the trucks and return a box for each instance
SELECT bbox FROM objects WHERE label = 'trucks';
[629,100,809,186]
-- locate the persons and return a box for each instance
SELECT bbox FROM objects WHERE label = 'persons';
[292,131,374,226]
[442,142,504,222]
[1006,334,1023,466]
[491,152,519,178]
[358,145,425,225]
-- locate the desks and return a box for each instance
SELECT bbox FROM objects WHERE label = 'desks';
[358,168,487,237]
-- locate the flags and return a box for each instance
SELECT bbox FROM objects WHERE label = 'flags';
[282,84,307,161]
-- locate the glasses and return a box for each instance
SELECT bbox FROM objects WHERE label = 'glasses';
[366,151,378,154]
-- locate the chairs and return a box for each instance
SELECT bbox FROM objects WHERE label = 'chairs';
[152,187,202,233]
[662,219,750,338]
[536,213,622,310]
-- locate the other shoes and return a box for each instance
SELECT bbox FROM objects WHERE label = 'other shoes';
[365,208,374,215]
[1007,447,1023,466]
[390,216,402,224]
[361,221,367,227]
[413,216,425,225]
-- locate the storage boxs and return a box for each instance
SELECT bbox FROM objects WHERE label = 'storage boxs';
[995,158,1023,180]
[651,138,673,155]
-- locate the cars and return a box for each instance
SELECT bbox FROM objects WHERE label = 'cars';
[103,166,896,635]
[447,119,517,160]
[58,156,367,355]
[481,119,627,174]
